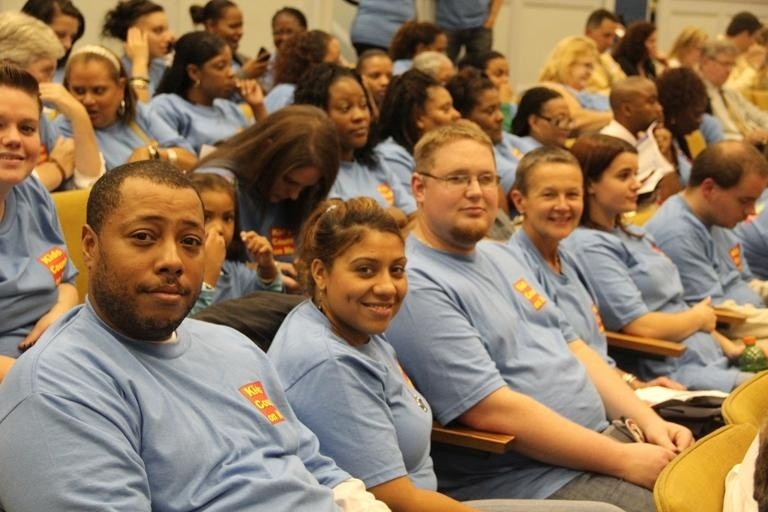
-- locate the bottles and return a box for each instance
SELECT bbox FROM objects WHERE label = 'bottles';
[739,335,767,376]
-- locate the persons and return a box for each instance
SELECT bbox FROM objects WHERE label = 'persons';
[1,1,767,510]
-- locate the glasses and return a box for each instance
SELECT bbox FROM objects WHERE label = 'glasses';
[418,171,501,188]
[539,113,571,126]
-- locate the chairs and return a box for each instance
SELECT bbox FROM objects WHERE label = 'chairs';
[720,369,767,436]
[39,187,90,305]
[652,419,760,512]
